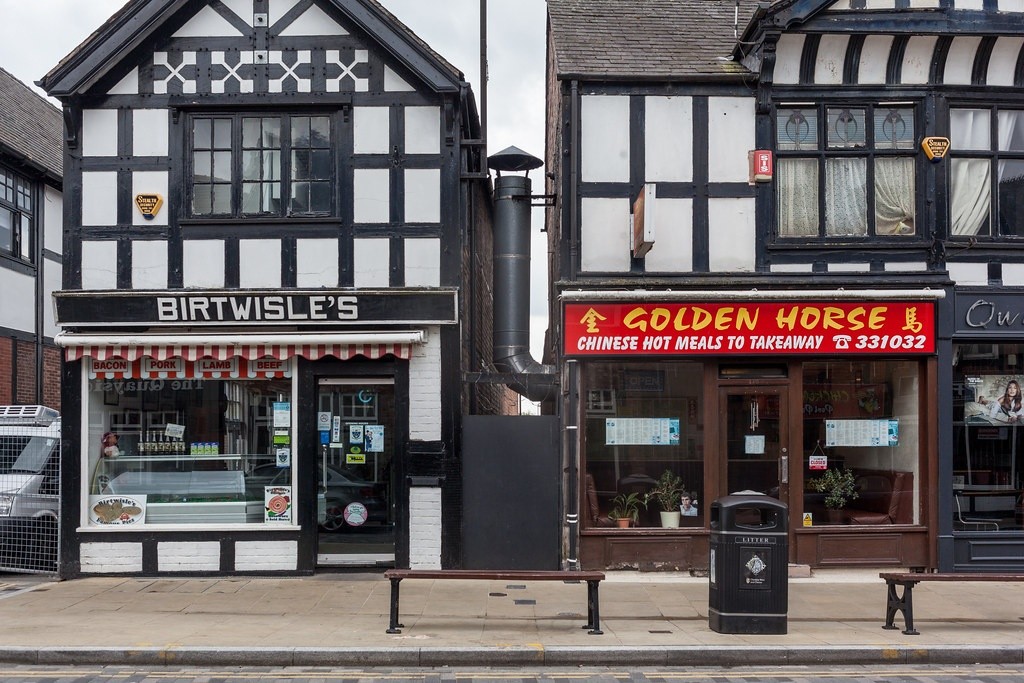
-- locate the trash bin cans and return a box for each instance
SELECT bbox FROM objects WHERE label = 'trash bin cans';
[709,491,790,635]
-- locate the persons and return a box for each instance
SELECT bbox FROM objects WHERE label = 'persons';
[979,380,1024,423]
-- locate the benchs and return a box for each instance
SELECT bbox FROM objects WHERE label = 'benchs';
[880,572,1024,635]
[384,569,605,635]
[585,470,640,527]
[804,467,912,525]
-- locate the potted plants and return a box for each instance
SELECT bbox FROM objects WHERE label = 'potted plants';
[608,492,648,528]
[647,469,687,530]
[810,468,860,525]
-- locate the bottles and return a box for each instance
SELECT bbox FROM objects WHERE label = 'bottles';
[178,438,184,455]
[171,437,178,455]
[282,445,290,449]
[158,431,164,455]
[145,431,151,456]
[273,445,279,455]
[164,436,171,455]
[151,431,158,456]
[138,431,145,456]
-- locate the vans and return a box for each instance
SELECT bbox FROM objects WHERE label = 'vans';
[0,405,62,572]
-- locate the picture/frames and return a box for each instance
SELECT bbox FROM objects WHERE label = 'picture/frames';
[103,379,187,412]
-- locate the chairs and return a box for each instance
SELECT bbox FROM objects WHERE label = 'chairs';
[955,495,1004,531]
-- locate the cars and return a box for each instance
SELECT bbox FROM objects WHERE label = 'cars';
[245,459,397,533]
